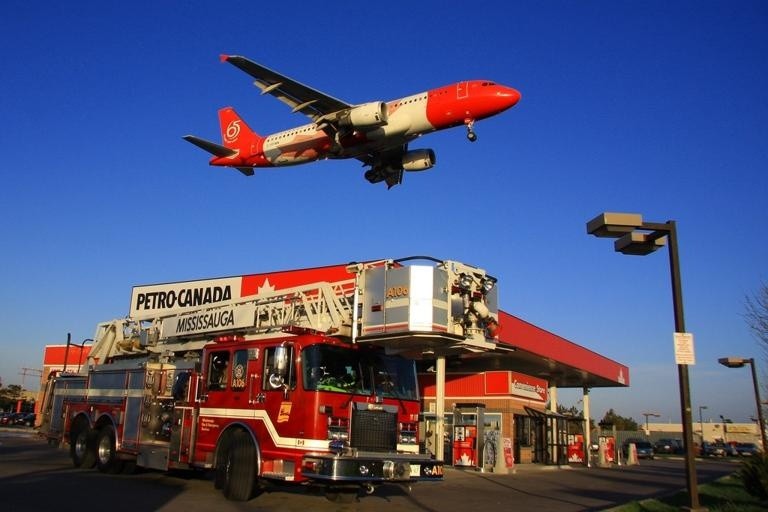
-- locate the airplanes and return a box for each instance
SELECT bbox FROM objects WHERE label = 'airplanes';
[182,53,521,191]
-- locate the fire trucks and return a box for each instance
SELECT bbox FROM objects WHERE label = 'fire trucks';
[40,256,499,501]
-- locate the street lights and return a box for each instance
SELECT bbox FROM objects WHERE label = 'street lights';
[699,406,707,432]
[642,413,660,430]
[586,211,708,512]
[718,357,768,449]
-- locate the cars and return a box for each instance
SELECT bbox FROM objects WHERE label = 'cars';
[620,437,763,458]
[0,412,35,426]
